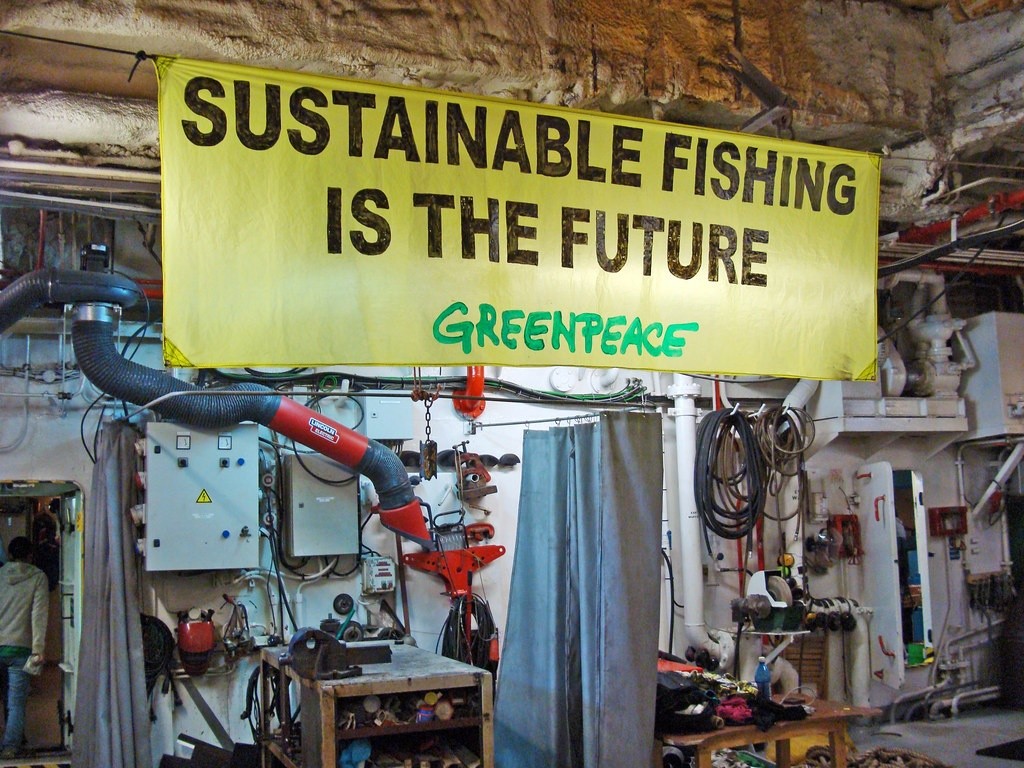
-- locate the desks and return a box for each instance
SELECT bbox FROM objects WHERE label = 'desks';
[259,644,494,768]
[662,688,884,768]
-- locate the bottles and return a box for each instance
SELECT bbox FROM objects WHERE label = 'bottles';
[754,656,772,701]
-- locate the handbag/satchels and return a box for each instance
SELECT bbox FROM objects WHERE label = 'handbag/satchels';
[653,669,719,734]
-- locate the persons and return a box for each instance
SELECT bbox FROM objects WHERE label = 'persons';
[895,506,914,653]
[0,536,49,760]
[0,514,59,593]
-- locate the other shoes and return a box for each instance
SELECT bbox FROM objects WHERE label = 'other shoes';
[0,746,33,759]
